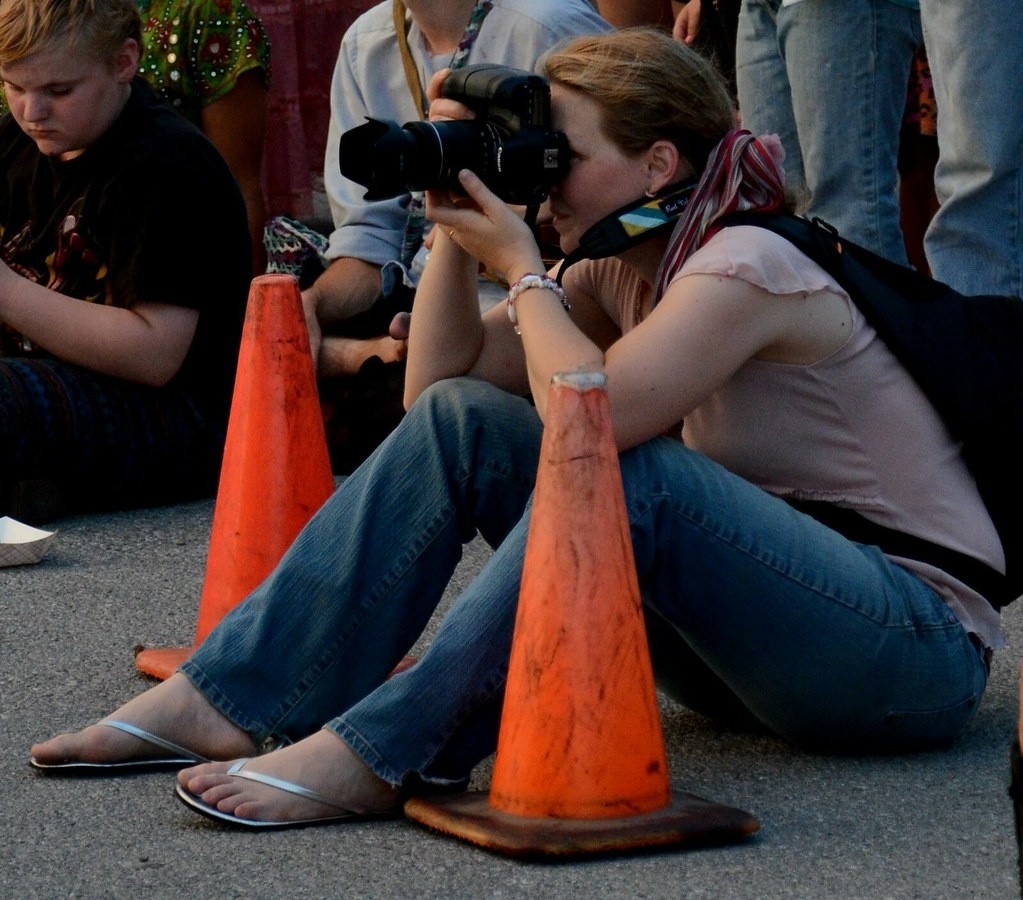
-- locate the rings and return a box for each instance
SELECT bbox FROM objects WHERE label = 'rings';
[449,231,454,241]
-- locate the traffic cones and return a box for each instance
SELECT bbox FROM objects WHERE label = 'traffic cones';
[404,370,767,862]
[134,274,419,688]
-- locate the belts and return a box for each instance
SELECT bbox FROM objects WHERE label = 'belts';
[968,631,993,676]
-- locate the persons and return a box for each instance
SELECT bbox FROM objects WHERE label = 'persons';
[672,0,921,267]
[0,0,252,526]
[921,0,1023,298]
[28,30,1006,830]
[302,0,627,473]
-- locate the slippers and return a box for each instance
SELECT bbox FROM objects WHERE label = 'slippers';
[177,759,365,826]
[30,720,211,766]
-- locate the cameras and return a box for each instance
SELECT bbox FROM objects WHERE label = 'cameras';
[338,62,574,205]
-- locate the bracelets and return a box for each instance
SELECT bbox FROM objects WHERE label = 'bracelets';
[507,274,572,335]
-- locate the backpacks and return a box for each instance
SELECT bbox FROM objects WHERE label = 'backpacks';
[711,209,1023,614]
